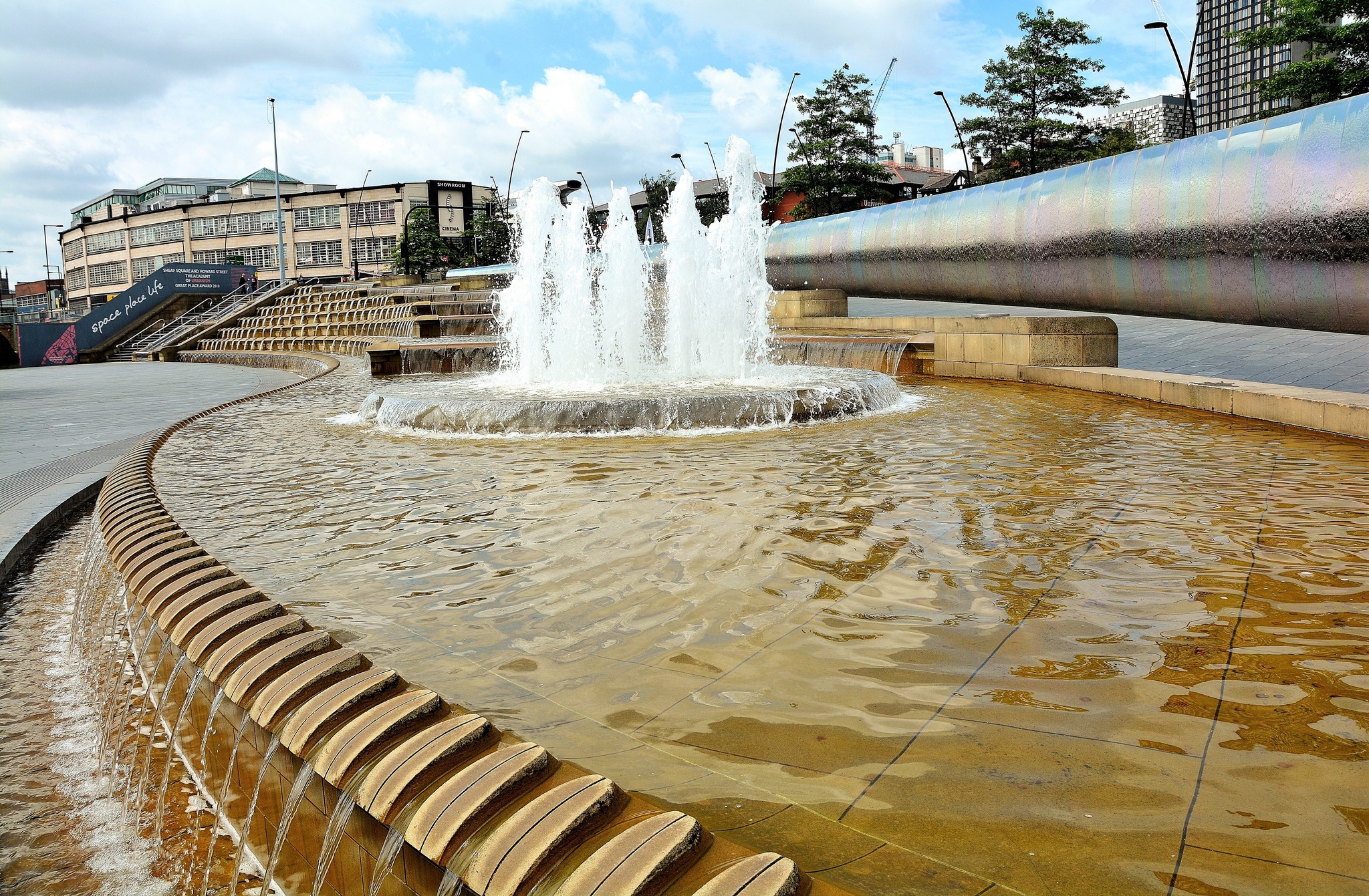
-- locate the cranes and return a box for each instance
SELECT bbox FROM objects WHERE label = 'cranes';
[860,55,898,181]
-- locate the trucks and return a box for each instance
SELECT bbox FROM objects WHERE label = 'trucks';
[348,274,382,282]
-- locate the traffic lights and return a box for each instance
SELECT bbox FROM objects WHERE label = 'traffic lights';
[490,202,496,216]
[475,237,483,254]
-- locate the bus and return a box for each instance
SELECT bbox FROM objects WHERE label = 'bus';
[302,276,347,284]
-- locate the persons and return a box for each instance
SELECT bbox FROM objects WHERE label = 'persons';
[449,262,469,270]
[239,273,250,295]
[298,273,304,283]
[250,274,257,292]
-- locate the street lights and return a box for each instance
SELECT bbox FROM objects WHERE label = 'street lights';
[490,131,530,262]
[267,98,287,288]
[771,72,818,225]
[704,142,724,209]
[43,225,66,320]
[576,172,597,226]
[225,197,238,264]
[355,170,379,281]
[169,238,186,264]
[0,251,14,315]
[932,90,971,188]
[1144,22,1198,139]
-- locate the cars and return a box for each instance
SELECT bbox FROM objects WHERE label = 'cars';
[57,314,81,319]
